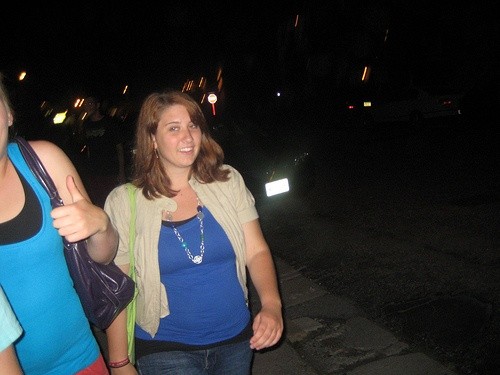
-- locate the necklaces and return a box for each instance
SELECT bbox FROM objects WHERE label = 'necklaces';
[160,185,205,265]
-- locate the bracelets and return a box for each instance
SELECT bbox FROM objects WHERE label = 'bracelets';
[108,358,130,368]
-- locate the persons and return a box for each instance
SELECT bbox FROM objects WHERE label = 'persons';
[99,90,285,375]
[0,86,118,375]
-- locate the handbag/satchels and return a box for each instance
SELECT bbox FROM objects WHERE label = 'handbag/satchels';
[17,138,135,331]
